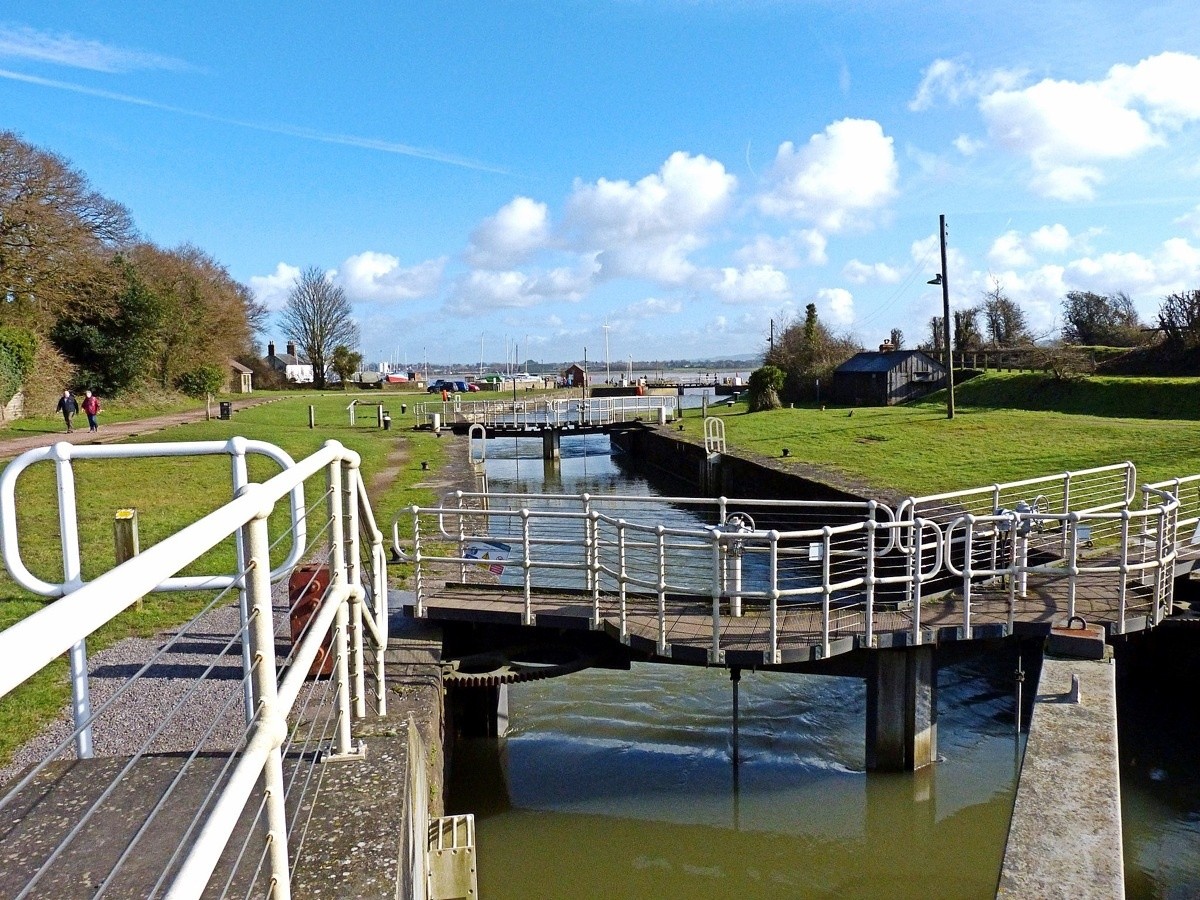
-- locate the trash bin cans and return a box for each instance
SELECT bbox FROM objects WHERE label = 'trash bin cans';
[220,401,233,421]
[287,563,334,681]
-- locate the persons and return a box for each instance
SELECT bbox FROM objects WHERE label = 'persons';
[706,372,710,383]
[644,374,648,384]
[81,389,102,433]
[713,371,719,383]
[563,376,567,387]
[56,389,80,434]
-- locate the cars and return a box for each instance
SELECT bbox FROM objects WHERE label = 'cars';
[468,383,480,392]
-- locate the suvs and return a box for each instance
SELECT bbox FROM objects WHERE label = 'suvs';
[454,382,469,393]
[426,381,459,393]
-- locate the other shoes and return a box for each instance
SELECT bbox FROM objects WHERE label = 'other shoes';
[67,425,97,434]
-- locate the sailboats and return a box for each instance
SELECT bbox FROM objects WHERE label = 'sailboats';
[467,332,543,383]
